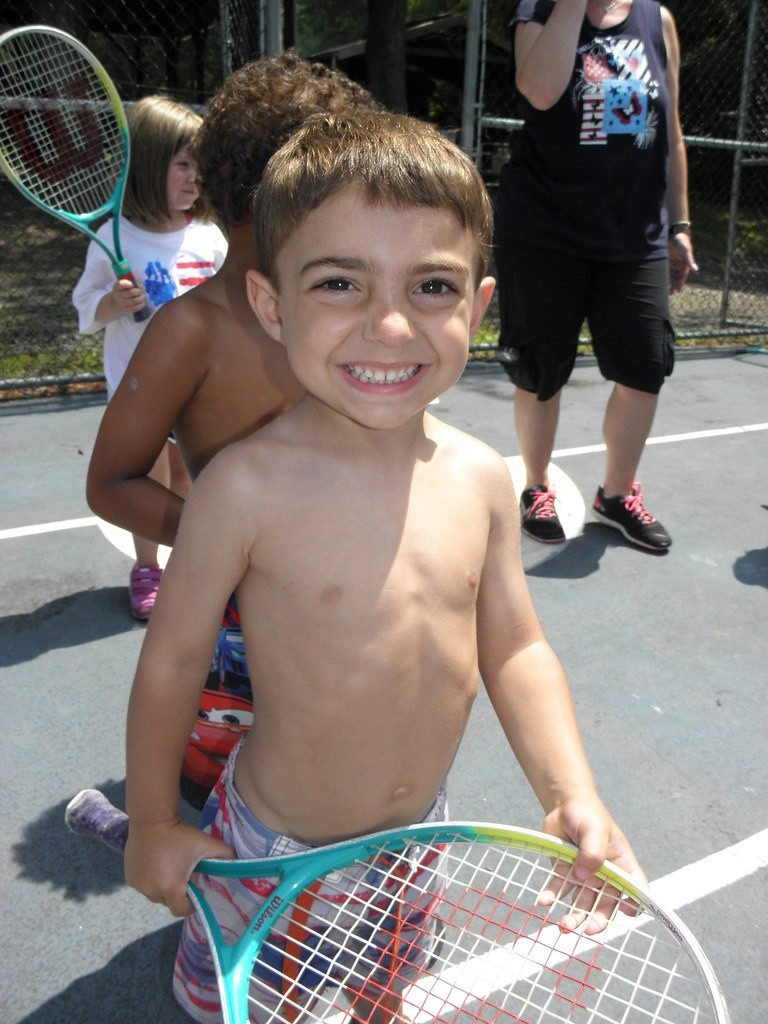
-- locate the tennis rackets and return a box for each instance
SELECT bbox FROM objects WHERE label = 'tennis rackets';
[65,785,739,1024]
[0,24,154,321]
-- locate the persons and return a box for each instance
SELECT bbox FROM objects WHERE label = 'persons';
[71,52,645,1024]
[491,0,700,553]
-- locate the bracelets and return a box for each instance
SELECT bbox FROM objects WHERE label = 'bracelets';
[669,220,691,234]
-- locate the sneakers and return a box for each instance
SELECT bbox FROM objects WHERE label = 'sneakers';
[592,480,674,553]
[520,484,566,542]
[128,562,165,621]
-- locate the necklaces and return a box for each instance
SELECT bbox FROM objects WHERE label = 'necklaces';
[590,0,618,14]
